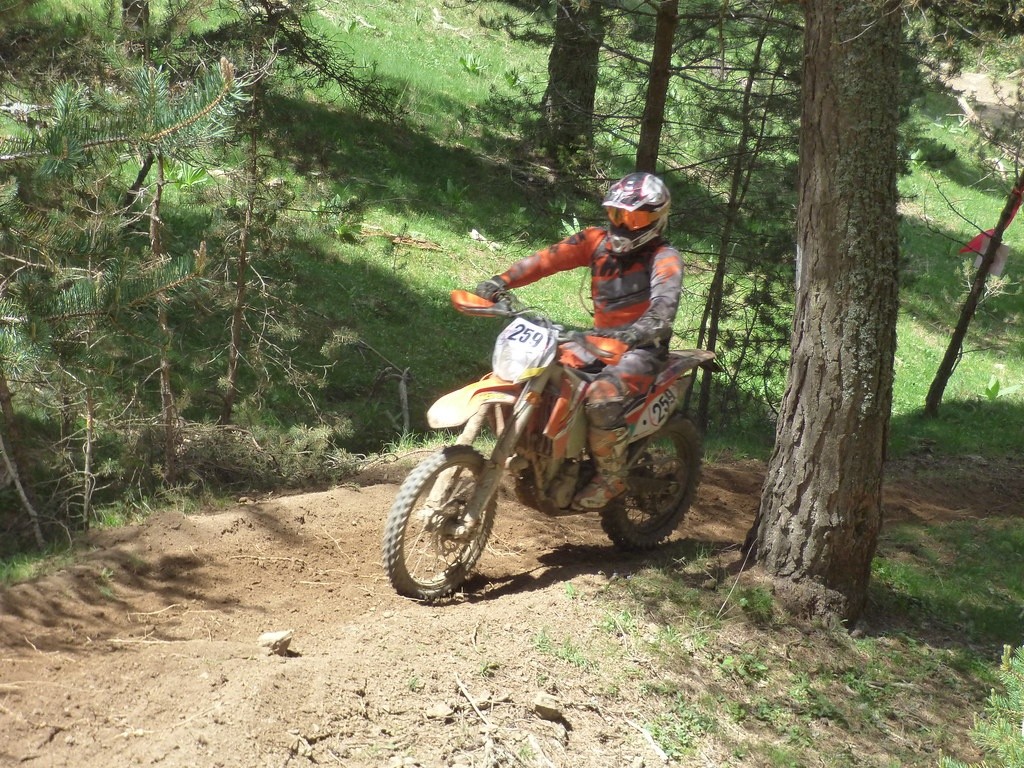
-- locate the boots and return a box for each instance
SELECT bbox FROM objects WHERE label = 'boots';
[570,426,631,511]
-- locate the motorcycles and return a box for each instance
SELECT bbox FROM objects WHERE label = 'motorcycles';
[380,287,727,600]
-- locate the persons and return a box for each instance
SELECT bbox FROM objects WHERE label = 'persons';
[476,172,684,511]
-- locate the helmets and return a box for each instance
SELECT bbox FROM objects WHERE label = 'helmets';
[600,172,672,255]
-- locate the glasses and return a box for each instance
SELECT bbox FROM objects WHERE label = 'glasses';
[607,205,658,231]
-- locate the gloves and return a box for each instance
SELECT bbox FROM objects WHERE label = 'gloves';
[474,275,507,301]
[603,327,639,352]
